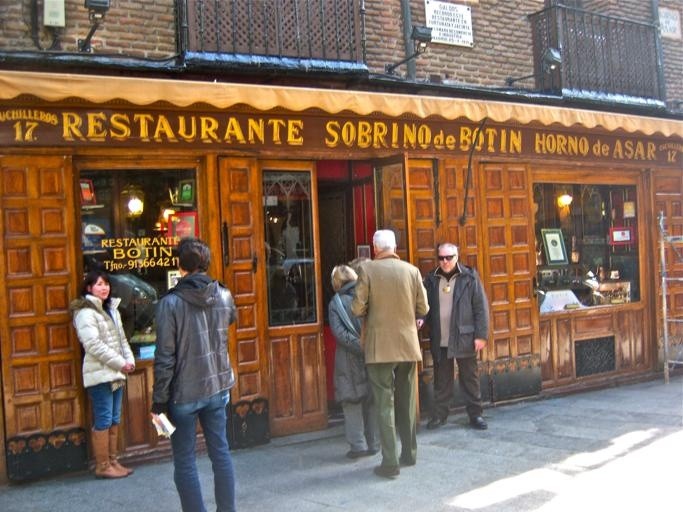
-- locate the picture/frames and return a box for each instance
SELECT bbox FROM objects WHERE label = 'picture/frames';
[168,270,181,290]
[357,244,370,261]
[541,228,569,266]
[177,180,195,203]
[608,227,636,245]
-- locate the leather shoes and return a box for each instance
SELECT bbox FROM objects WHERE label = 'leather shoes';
[374,464,400,476]
[471,414,488,430]
[427,415,448,429]
[346,445,379,458]
[398,455,420,465]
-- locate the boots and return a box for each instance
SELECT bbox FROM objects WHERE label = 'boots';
[91,421,133,479]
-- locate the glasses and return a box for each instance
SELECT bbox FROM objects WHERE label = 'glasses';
[439,254,458,261]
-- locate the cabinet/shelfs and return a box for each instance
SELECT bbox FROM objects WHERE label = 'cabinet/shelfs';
[658,209,682,384]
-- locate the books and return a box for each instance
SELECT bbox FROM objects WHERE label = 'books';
[152,413,176,439]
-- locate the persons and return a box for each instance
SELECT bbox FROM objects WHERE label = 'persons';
[70,270,136,479]
[269,263,311,322]
[328,230,490,477]
[149,239,238,512]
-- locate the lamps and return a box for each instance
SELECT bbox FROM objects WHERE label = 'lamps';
[119,185,145,218]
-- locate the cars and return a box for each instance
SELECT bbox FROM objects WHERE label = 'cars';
[80,234,158,329]
[282,259,314,310]
